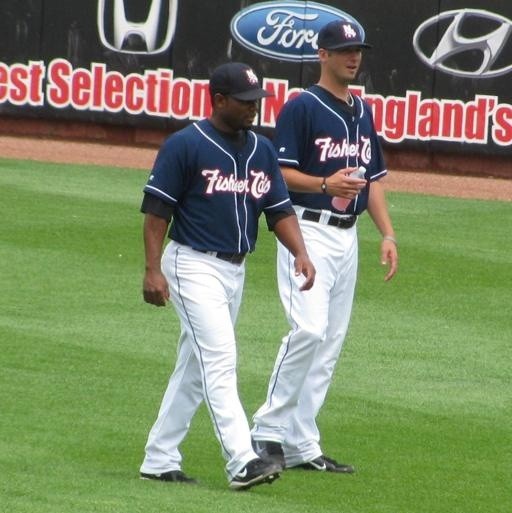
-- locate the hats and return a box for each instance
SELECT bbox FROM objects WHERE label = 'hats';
[318,20,373,51]
[210,62,275,101]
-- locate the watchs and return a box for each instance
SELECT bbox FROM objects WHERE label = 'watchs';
[320,176,329,195]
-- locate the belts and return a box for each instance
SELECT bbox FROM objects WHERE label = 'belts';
[302,212,361,228]
[200,249,247,263]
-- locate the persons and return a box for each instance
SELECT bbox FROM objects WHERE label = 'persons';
[136,58,318,495]
[245,17,402,475]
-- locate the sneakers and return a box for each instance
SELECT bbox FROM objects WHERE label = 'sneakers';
[231,458,282,494]
[138,469,199,485]
[298,456,356,475]
[248,435,290,473]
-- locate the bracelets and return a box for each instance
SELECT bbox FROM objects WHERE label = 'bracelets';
[384,233,399,248]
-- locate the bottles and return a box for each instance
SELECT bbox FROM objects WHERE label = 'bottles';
[331,164,367,211]
